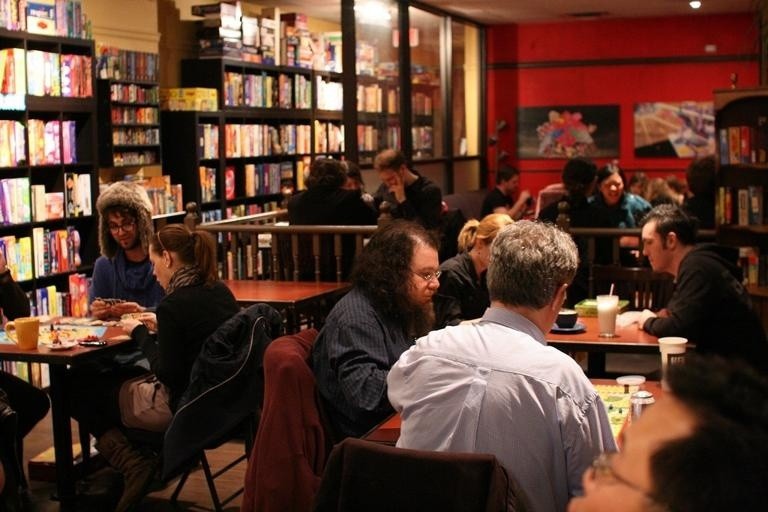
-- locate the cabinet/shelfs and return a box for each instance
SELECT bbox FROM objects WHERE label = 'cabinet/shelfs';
[185,61,438,224]
[712,90,768,327]
[97,47,182,233]
[2,31,99,390]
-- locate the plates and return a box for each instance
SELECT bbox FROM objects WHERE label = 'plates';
[549,321,585,333]
[46,341,77,351]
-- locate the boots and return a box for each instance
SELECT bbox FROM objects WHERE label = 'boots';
[94,428,154,512]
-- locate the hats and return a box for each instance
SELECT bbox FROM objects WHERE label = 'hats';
[94,180,154,258]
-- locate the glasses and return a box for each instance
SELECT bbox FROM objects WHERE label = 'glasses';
[107,222,135,234]
[413,271,441,279]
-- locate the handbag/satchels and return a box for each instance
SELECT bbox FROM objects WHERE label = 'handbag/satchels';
[51,474,115,511]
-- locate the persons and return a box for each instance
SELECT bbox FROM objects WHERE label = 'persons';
[587,161,654,229]
[88,181,165,384]
[480,165,532,222]
[1,249,49,511]
[627,171,690,209]
[387,220,619,512]
[537,155,619,310]
[61,225,240,512]
[307,218,442,457]
[341,161,373,201]
[362,149,439,231]
[436,213,513,330]
[567,352,766,512]
[638,202,768,366]
[288,157,378,284]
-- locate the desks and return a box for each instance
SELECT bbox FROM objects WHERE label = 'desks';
[0,315,145,501]
[542,304,678,373]
[222,277,351,335]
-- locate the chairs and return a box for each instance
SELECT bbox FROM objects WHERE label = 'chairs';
[249,326,344,510]
[310,438,540,510]
[159,316,277,512]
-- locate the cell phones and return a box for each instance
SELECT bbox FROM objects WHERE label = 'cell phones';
[79,340,107,346]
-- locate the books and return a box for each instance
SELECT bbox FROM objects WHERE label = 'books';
[715,93,767,293]
[191,1,344,223]
[96,47,183,217]
[1,225,80,282]
[29,274,92,314]
[357,45,433,163]
[1,119,76,168]
[1,0,92,39]
[1,172,92,226]
[2,48,92,110]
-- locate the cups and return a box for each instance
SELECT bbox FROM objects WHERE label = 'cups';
[595,294,618,339]
[553,308,578,328]
[4,316,41,350]
[656,336,689,394]
